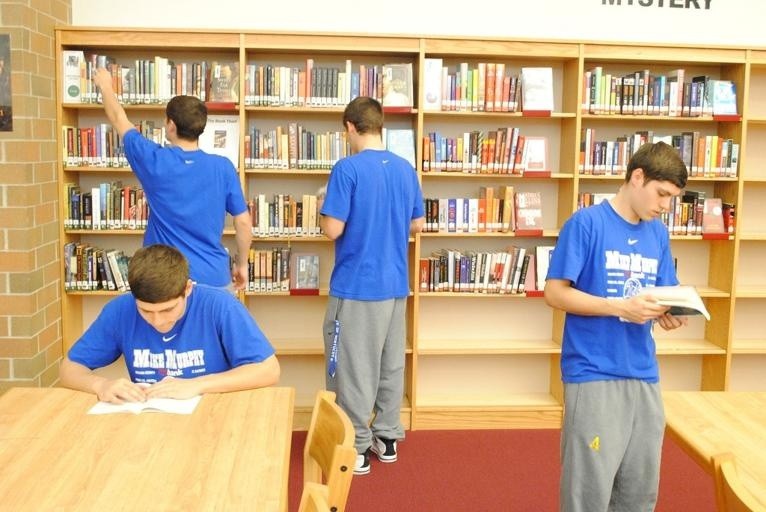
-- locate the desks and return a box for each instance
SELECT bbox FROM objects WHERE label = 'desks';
[665,390,765,511]
[1,386,296,512]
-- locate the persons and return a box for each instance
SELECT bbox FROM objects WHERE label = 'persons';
[93,68,253,296]
[543,141,689,512]
[57,244,281,405]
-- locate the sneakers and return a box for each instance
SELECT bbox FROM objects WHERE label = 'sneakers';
[370,435,398,464]
[354,449,373,476]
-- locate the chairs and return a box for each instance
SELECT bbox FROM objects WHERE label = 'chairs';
[712,453,766,512]
[296,386,358,512]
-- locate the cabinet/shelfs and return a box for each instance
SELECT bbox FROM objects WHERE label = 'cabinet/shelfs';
[52,25,766,433]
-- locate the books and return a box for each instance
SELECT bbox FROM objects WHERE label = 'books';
[62,50,92,105]
[526,136,548,171]
[583,68,738,118]
[662,191,736,234]
[93,55,240,103]
[246,123,352,170]
[421,185,518,233]
[94,248,131,293]
[85,381,202,416]
[197,115,240,167]
[297,255,320,289]
[64,182,92,230]
[419,240,554,292]
[636,283,714,324]
[578,190,616,210]
[95,122,167,168]
[246,59,412,106]
[93,181,151,230]
[387,129,417,171]
[523,67,554,110]
[516,192,542,230]
[246,192,325,238]
[249,250,291,294]
[425,58,522,113]
[422,127,526,175]
[576,127,740,178]
[63,125,93,168]
[63,240,93,293]
[382,128,386,151]
